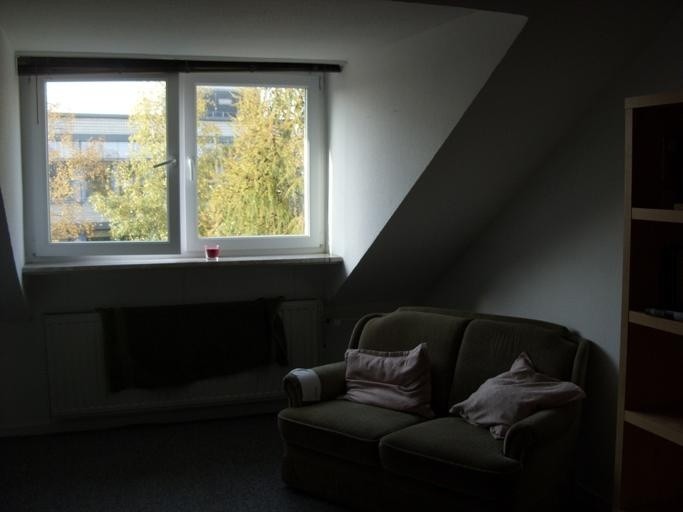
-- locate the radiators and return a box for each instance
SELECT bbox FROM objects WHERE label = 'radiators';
[46,297,326,419]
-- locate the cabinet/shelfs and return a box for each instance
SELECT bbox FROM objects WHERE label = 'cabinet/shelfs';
[611,86,683,511]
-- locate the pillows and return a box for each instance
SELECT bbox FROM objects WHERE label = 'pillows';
[343,341,436,421]
[449,351,586,442]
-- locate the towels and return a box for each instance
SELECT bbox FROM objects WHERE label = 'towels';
[95,301,290,394]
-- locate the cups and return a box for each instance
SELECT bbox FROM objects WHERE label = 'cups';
[204,244,218,262]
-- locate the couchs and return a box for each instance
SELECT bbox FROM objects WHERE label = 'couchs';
[275,303,599,512]
